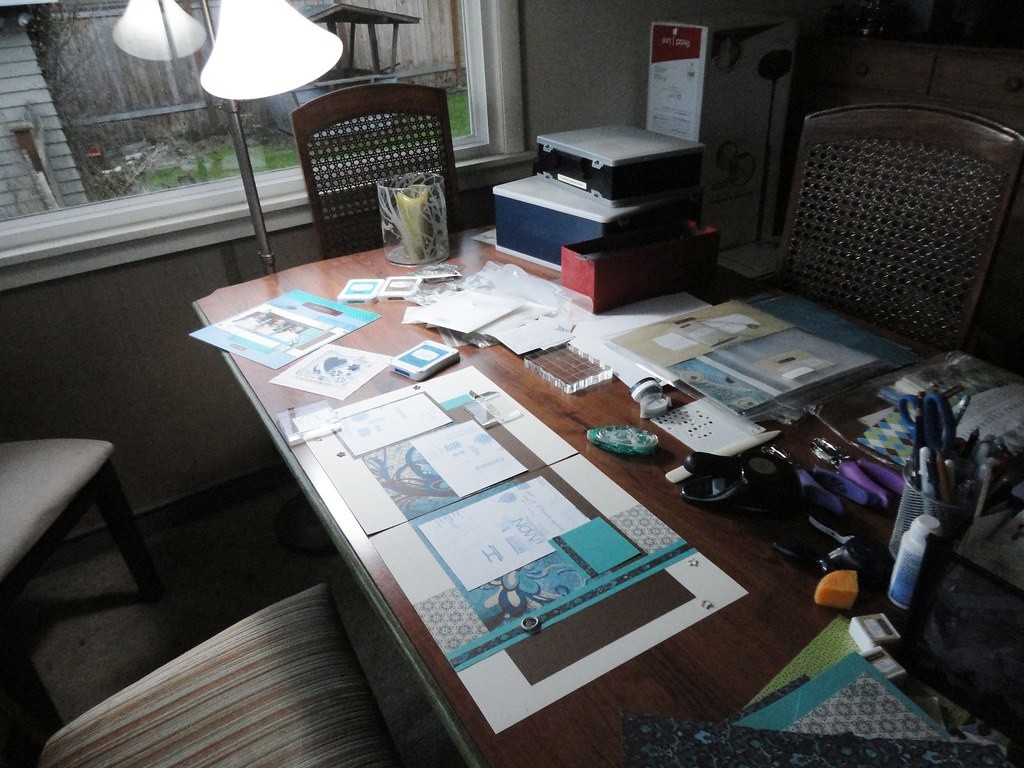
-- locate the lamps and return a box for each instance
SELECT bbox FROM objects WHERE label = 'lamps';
[200,0,342,276]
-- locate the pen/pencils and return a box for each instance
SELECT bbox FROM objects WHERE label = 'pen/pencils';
[899,390,1018,525]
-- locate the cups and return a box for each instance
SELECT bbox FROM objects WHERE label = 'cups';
[375,172,451,269]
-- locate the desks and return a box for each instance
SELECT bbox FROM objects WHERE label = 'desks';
[193,220,942,768]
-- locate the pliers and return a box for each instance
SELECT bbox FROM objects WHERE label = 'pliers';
[770,514,859,582]
[809,435,906,510]
[760,443,879,517]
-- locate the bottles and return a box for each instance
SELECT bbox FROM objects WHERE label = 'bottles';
[887,512,941,609]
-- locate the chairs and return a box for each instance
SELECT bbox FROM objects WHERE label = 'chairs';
[0,437,163,735]
[290,83,466,260]
[741,102,1024,366]
[34,582,401,768]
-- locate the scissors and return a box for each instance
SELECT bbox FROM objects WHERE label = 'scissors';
[895,391,957,454]
